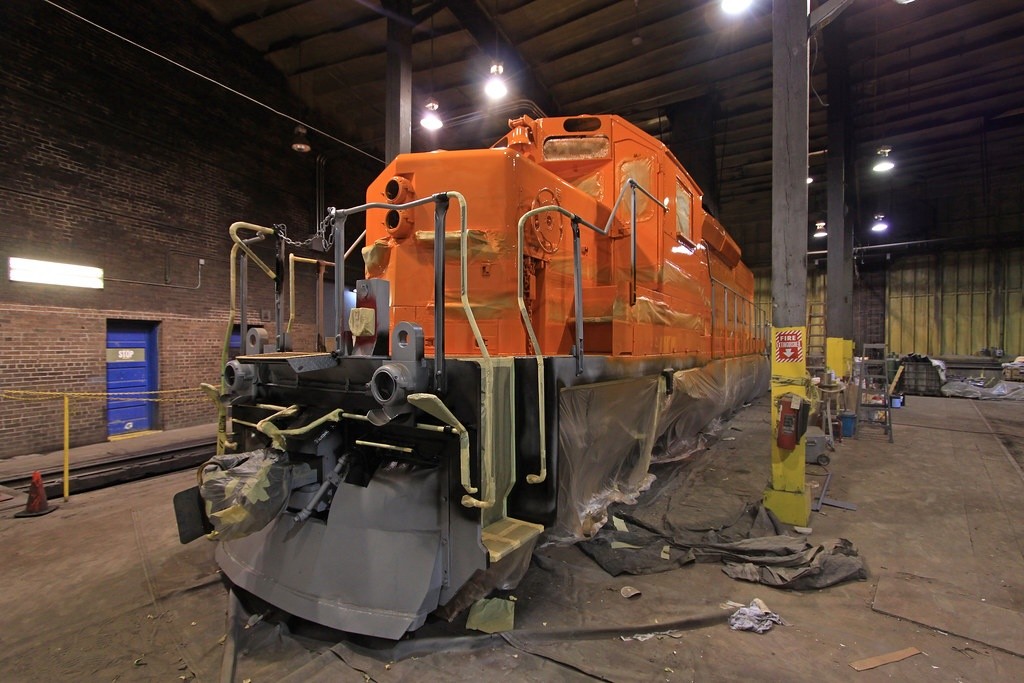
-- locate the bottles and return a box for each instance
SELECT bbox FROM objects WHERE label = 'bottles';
[831,369,835,380]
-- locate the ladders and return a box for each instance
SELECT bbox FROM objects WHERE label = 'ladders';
[854,343,894,443]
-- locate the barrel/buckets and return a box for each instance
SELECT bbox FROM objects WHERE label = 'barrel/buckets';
[841,413,855,437]
[890,396,901,409]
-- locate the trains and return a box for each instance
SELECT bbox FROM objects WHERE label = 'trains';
[172,115,770,644]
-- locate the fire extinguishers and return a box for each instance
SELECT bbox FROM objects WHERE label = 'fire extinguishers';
[775,392,802,449]
